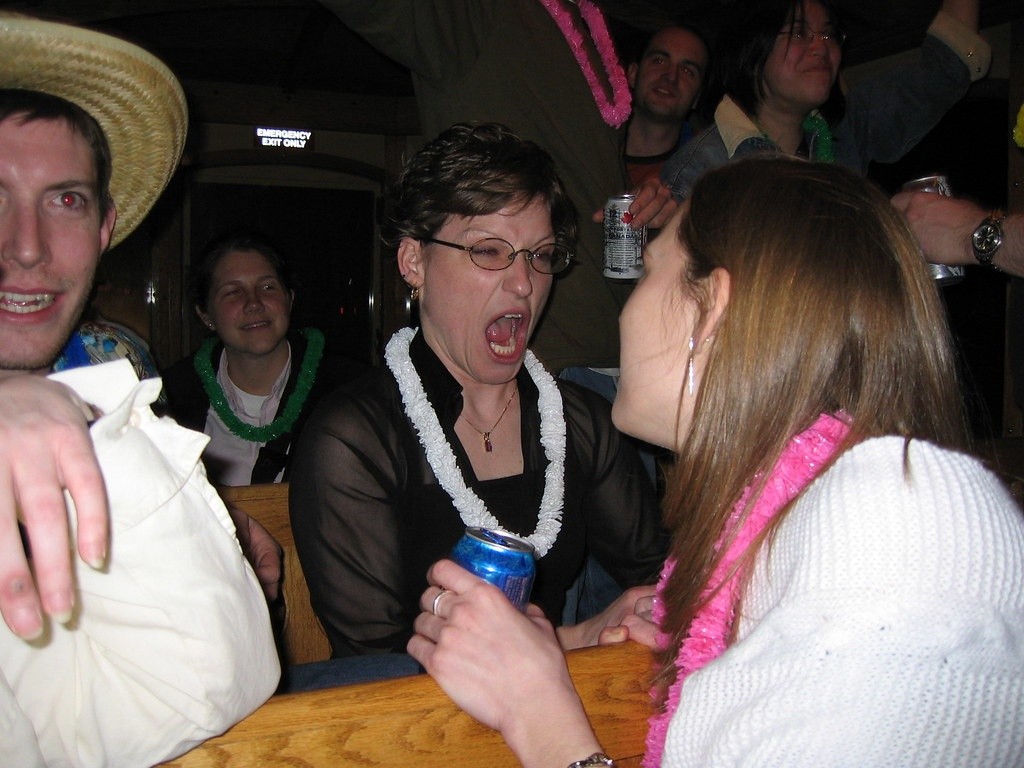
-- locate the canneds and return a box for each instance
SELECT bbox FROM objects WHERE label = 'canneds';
[904,175,965,285]
[603,195,648,279]
[450,526,536,615]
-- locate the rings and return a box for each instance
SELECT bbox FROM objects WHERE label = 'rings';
[650,595,659,607]
[431,588,454,615]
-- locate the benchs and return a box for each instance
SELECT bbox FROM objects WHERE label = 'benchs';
[220,482,335,664]
[155,639,676,768]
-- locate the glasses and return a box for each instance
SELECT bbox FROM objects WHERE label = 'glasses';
[778,26,846,48]
[414,234,577,275]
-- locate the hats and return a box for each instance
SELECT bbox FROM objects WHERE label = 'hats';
[0,11,189,254]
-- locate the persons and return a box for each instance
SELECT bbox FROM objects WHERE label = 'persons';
[0,0,1023,768]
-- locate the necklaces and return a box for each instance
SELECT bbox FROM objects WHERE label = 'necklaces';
[384,324,567,559]
[459,380,520,454]
[640,404,854,768]
[542,1,630,127]
[745,109,836,161]
[194,326,323,444]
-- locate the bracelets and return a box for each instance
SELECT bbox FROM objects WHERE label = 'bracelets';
[567,753,615,768]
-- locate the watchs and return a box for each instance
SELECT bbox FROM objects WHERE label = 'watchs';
[970,206,1013,273]
